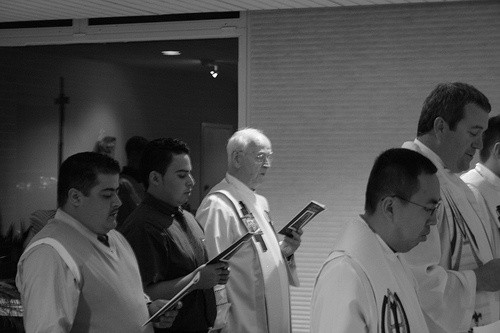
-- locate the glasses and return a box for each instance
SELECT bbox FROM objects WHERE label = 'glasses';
[382,194,441,217]
[238,149,273,163]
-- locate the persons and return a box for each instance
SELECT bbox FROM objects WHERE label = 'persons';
[195,128,302,333]
[15,133,231,333]
[310,148,443,333]
[397,80,500,333]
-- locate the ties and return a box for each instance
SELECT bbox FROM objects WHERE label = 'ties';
[99,234,111,248]
[176,213,214,330]
